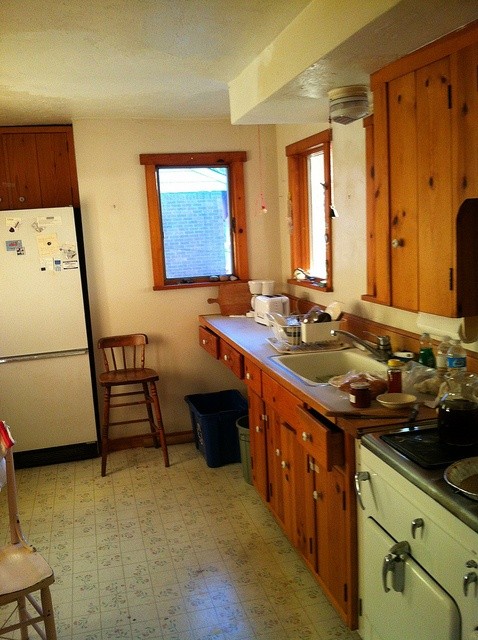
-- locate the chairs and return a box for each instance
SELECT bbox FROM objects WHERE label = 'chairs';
[0,419,57,640]
[98,333,170,477]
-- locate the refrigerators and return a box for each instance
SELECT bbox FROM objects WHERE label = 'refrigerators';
[1,206,99,453]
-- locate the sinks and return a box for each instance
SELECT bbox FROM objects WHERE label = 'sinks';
[268,348,391,386]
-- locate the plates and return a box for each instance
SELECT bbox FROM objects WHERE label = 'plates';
[329,375,347,387]
[443,454,478,499]
[377,392,418,409]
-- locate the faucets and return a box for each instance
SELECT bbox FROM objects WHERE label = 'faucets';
[331,329,392,357]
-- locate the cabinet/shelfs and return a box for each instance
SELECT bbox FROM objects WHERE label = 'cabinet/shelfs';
[295,403,355,632]
[244,365,269,505]
[369,21,478,318]
[198,314,243,381]
[261,371,303,552]
[0,125,80,210]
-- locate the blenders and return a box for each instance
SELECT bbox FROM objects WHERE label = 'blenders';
[247,295,255,317]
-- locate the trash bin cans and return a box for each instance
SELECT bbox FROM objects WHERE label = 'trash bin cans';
[184,389,248,468]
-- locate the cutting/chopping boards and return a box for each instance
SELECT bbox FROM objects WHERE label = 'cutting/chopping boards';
[208,280,251,316]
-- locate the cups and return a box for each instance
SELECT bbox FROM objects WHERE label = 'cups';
[247,280,260,293]
[261,280,274,296]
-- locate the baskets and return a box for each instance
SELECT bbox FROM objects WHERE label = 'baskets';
[267,313,349,351]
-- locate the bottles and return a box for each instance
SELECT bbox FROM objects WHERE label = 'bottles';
[437,370,478,447]
[419,333,433,367]
[387,359,402,392]
[435,336,450,373]
[447,340,468,372]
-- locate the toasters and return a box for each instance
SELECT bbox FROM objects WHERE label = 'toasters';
[253,294,291,326]
